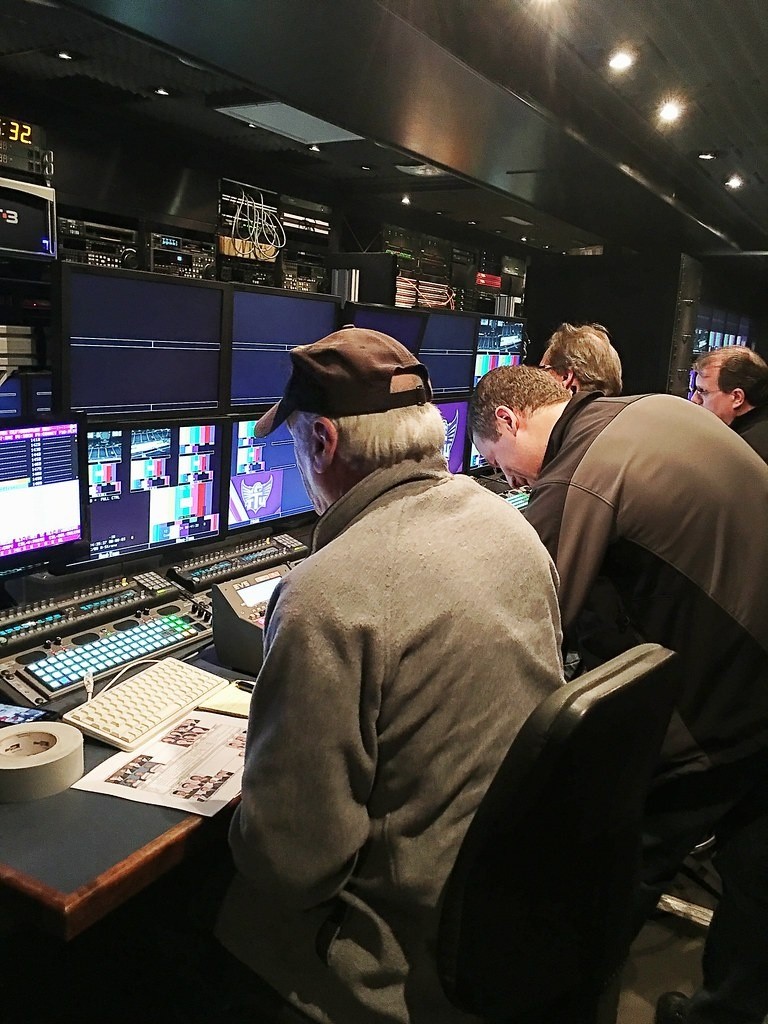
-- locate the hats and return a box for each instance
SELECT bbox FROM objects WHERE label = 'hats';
[254,324,435,439]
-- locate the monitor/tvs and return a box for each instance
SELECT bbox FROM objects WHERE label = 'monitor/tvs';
[0,414,87,574]
[432,395,470,475]
[467,440,502,475]
[79,416,235,568]
[221,412,316,534]
[46,260,528,417]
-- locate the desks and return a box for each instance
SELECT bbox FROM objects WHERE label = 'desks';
[0,638,259,943]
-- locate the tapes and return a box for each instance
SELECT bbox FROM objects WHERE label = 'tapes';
[0,721,84,804]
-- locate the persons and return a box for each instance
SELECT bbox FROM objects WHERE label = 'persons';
[95,323,569,1024]
[691,345,768,466]
[539,324,623,399]
[109,719,247,802]
[466,365,768,1024]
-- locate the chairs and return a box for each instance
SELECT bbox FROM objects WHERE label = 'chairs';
[153,641,682,1024]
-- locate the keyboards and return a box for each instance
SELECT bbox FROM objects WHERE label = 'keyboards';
[62,657,229,751]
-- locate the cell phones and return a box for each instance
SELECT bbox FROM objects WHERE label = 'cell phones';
[0,704,60,730]
